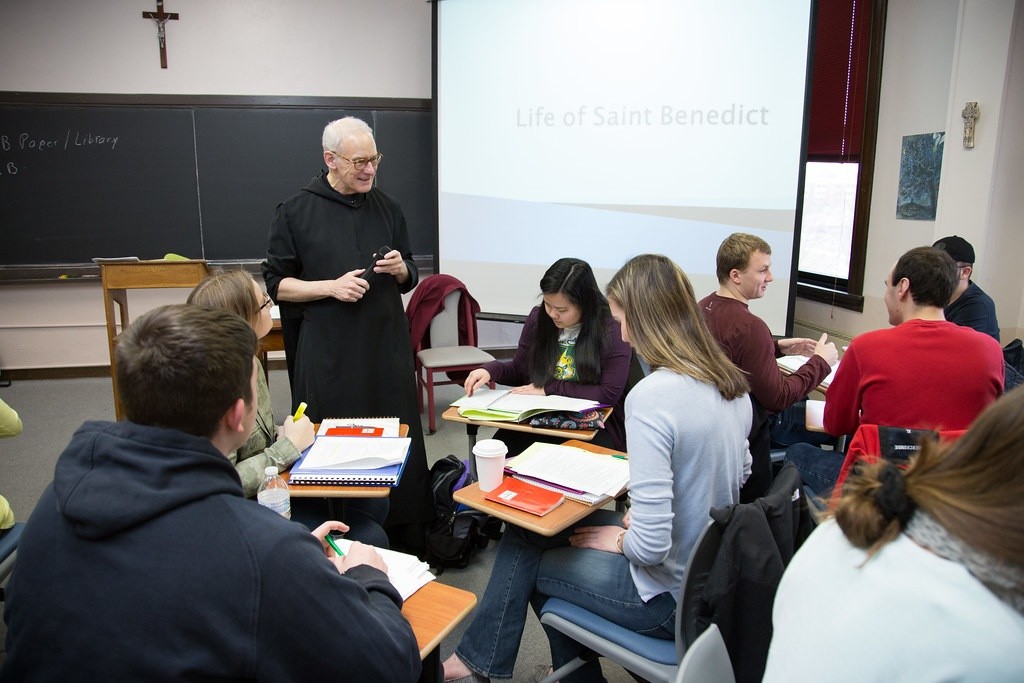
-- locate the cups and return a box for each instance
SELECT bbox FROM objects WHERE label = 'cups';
[471,439,509,493]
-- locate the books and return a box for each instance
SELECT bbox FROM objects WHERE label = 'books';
[290,417,412,487]
[503,441,630,505]
[777,356,840,388]
[485,477,565,516]
[449,389,604,423]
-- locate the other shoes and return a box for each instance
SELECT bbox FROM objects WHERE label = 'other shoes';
[532,664,552,683]
[445,670,491,683]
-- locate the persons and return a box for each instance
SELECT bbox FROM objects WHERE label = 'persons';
[0,303,423,683]
[443,255,752,683]
[696,233,838,449]
[464,258,632,456]
[188,272,392,549]
[782,247,1005,504]
[761,386,1024,683]
[933,235,999,344]
[264,117,435,549]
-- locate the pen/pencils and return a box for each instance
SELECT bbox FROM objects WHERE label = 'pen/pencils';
[325,535,343,556]
[613,455,628,461]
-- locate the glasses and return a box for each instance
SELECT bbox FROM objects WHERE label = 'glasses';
[332,150,383,170]
[254,291,275,315]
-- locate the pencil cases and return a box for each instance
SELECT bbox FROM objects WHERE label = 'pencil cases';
[529,409,606,431]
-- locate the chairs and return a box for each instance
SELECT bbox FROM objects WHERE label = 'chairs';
[536,462,799,683]
[410,275,495,437]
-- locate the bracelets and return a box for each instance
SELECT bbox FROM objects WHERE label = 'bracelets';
[616,530,628,553]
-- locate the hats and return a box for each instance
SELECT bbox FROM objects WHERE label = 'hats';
[932,236,974,264]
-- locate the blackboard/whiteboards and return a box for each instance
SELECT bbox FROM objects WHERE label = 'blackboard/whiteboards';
[0,90,438,283]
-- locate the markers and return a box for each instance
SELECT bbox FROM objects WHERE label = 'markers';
[293,402,307,422]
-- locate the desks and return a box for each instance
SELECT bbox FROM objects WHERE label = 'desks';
[775,355,846,454]
[266,422,409,497]
[443,389,614,441]
[400,581,478,664]
[452,439,635,537]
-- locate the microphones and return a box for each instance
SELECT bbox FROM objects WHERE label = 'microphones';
[358,246,392,287]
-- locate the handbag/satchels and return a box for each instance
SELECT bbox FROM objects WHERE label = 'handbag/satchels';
[529,407,606,431]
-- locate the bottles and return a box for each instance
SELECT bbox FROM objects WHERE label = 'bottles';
[257,466,291,521]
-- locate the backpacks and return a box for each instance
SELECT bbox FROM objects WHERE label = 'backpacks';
[422,454,491,570]
